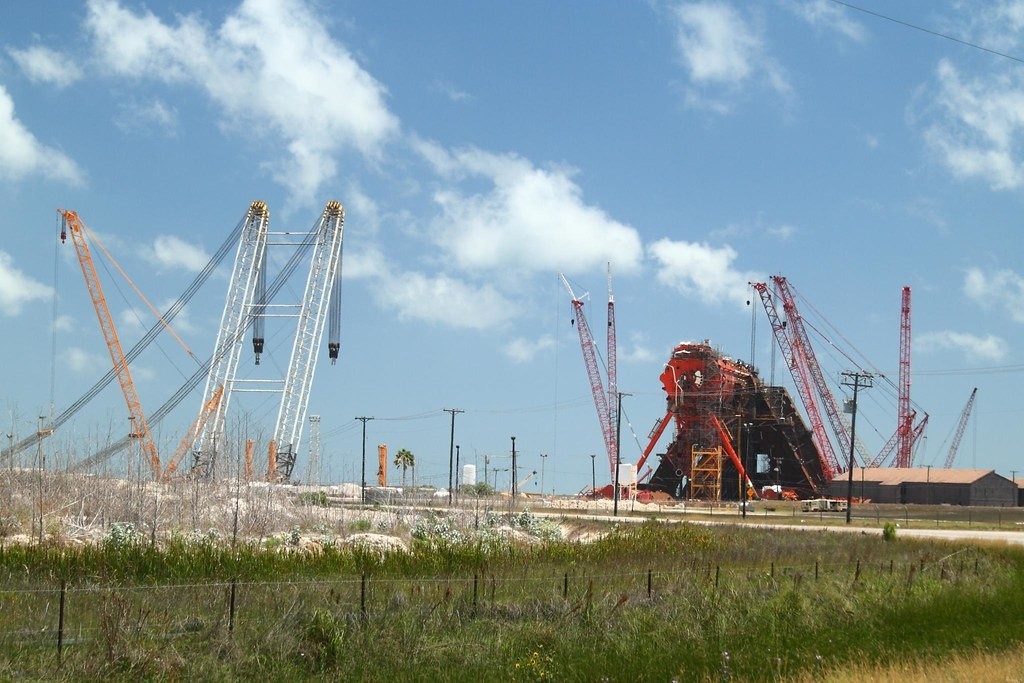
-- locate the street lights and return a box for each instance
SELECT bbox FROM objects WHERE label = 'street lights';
[510,436,517,497]
[455,445,461,491]
[591,454,598,492]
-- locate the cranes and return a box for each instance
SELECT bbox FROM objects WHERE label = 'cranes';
[56,197,343,485]
[557,259,624,495]
[747,273,980,479]
